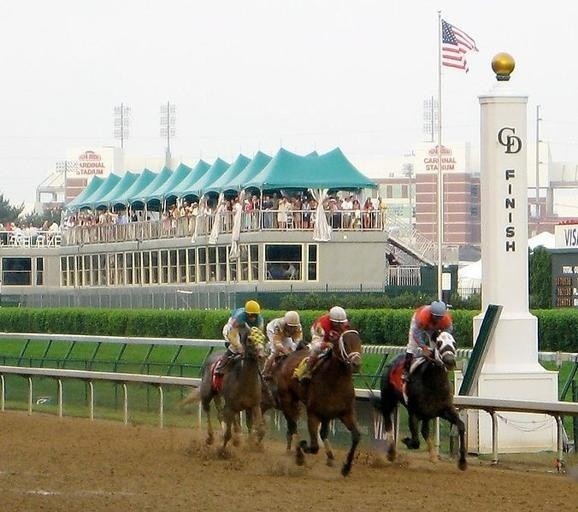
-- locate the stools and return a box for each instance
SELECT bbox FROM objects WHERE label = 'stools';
[0,226,61,247]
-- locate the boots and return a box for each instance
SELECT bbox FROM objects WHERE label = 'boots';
[299,354,318,385]
[261,357,274,379]
[400,352,413,381]
[213,347,234,376]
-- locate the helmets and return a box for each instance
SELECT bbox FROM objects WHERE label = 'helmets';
[328,306,347,323]
[429,299,447,317]
[283,309,301,327]
[244,300,261,316]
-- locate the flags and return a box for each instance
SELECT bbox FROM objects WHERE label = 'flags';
[441,19,479,74]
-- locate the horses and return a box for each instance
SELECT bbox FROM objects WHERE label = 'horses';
[365,327,468,470]
[275,320,363,476]
[175,321,265,457]
[245,340,306,457]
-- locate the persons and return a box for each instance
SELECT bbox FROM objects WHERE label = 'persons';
[299,306,349,387]
[260,310,303,379]
[1,195,386,246]
[210,300,264,376]
[400,300,454,384]
